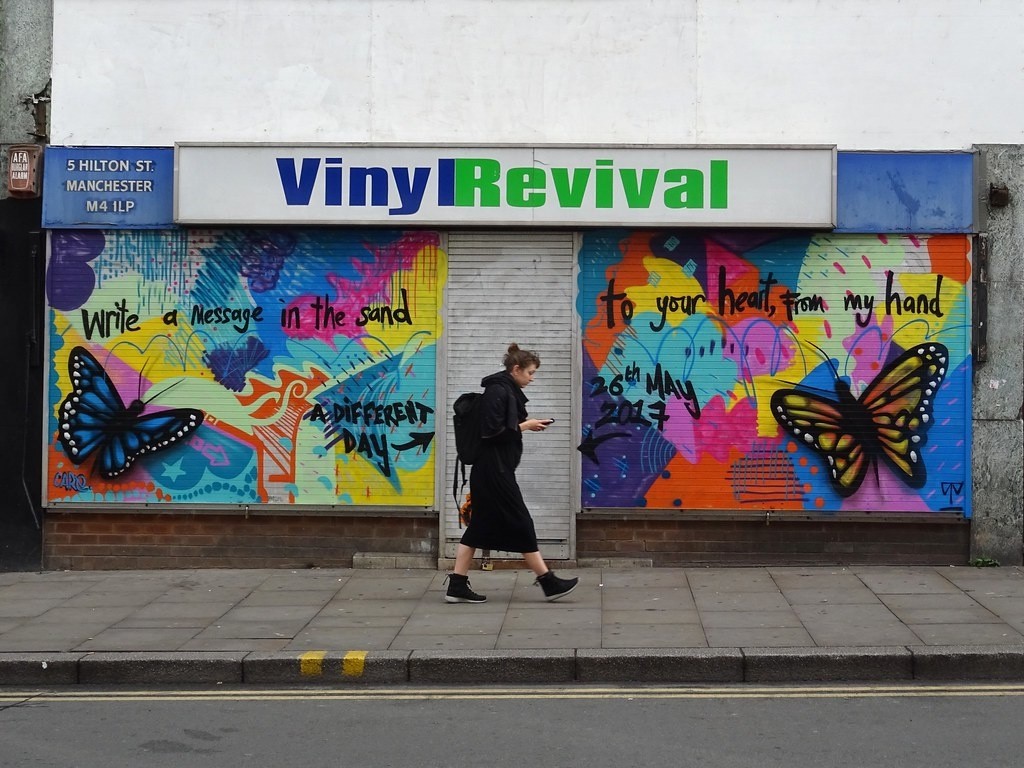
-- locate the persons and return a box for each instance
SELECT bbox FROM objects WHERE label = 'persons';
[443,341,581,604]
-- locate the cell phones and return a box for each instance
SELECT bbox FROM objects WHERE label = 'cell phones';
[542,418,555,426]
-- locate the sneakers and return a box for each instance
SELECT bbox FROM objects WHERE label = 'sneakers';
[446,573,487,603]
[537,569,581,602]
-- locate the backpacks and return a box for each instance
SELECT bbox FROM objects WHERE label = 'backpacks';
[453,382,515,465]
